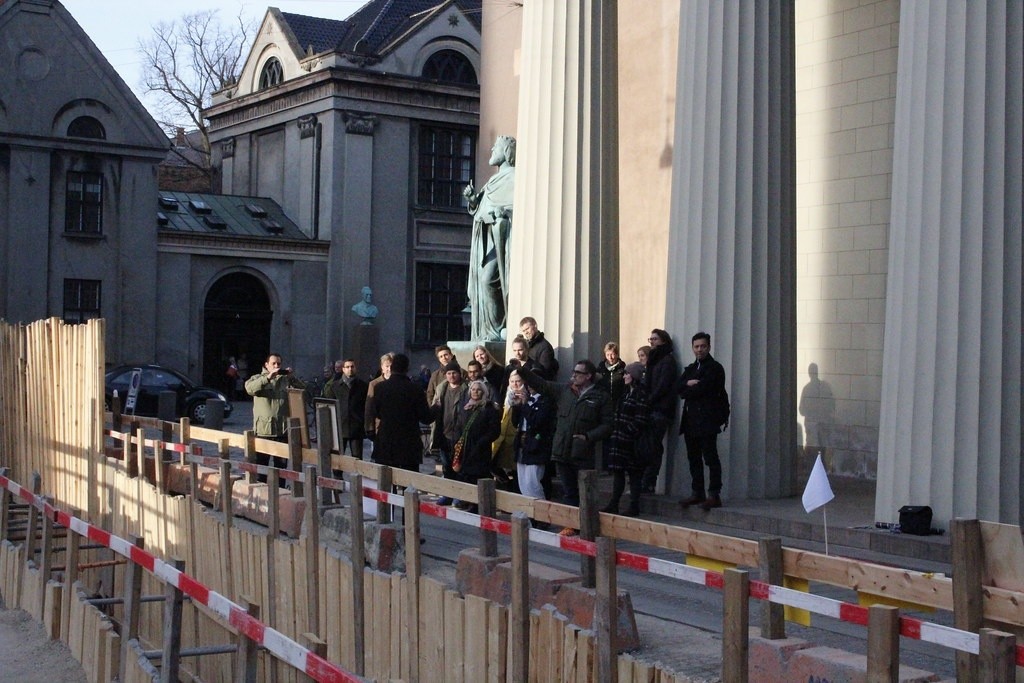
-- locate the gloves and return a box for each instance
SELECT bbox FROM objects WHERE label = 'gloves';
[367,430,377,442]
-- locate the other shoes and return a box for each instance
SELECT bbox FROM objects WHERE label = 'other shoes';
[598,503,620,515]
[639,485,655,496]
[436,496,453,505]
[455,500,465,506]
[620,506,640,518]
[698,495,721,509]
[558,529,579,536]
[679,494,707,506]
[460,504,478,514]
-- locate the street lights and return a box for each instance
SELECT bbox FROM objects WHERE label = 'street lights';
[460,300,472,342]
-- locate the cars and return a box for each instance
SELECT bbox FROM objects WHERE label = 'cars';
[106,360,234,426]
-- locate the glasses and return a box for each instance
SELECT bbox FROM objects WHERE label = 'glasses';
[571,370,588,376]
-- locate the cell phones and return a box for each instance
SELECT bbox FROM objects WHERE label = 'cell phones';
[278,370,289,375]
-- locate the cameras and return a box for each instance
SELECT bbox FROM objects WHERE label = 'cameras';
[514,393,522,396]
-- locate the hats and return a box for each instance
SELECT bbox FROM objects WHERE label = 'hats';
[444,356,460,372]
[624,362,644,382]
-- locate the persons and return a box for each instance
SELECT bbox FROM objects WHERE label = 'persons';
[221,351,248,402]
[245,315,731,538]
[352,285,379,325]
[462,134,516,341]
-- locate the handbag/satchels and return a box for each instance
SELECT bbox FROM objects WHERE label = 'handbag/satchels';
[899,506,933,536]
[699,361,730,426]
[452,436,465,472]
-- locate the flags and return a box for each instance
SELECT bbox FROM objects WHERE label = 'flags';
[802,455,836,514]
